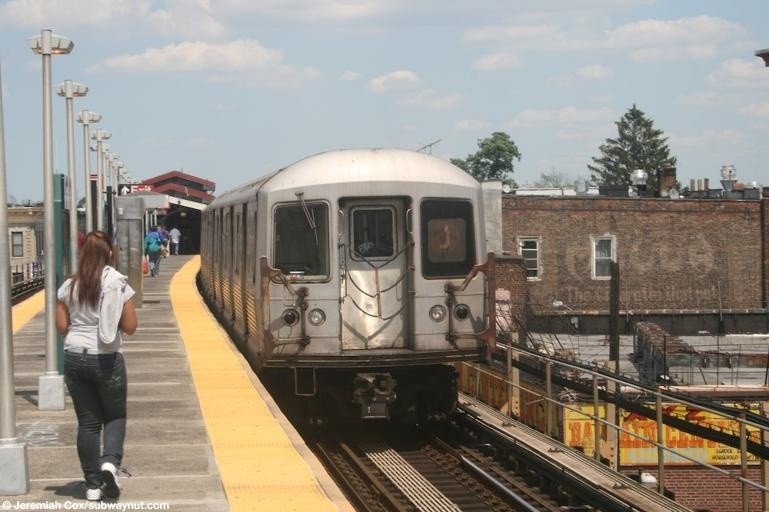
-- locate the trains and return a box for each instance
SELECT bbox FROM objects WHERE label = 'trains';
[199,149,496,446]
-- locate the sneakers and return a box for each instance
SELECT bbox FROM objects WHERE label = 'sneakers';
[100,462,122,498]
[86,487,106,501]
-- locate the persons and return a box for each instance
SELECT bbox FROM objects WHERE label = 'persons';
[53,230,141,504]
[156,222,170,258]
[143,224,168,277]
[167,225,183,255]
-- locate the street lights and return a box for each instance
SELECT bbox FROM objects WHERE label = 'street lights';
[75,110,102,234]
[56,79,89,279]
[29,28,75,409]
[90,130,129,233]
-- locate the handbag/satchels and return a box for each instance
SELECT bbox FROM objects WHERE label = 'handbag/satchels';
[144,261,149,274]
